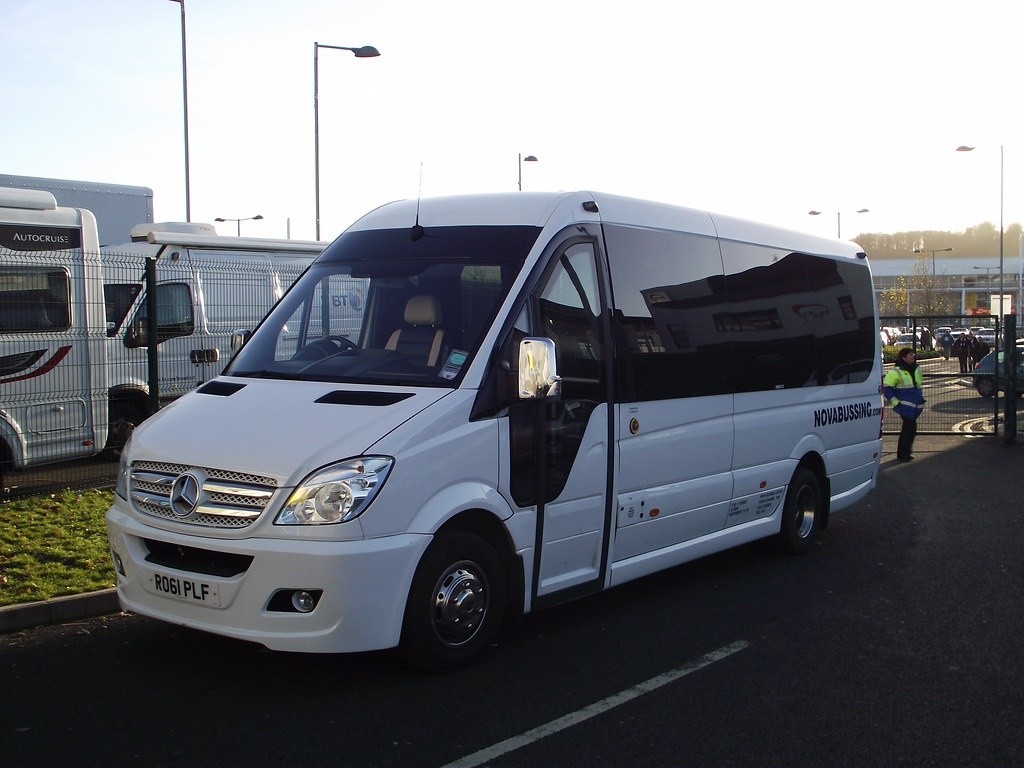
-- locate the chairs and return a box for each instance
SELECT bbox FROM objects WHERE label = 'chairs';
[384,294,451,368]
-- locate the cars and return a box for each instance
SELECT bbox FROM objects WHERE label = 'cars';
[975,339,1024,398]
[879,322,1024,350]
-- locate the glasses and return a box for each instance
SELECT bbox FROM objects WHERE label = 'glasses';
[907,354,915,357]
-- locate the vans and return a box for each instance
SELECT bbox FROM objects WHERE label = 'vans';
[106,190,884,673]
[0,184,371,471]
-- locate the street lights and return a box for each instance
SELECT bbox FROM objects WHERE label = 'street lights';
[973,266,1002,322]
[314,41,381,238]
[914,248,954,302]
[519,153,537,193]
[955,145,1004,339]
[215,215,263,237]
[808,209,870,241]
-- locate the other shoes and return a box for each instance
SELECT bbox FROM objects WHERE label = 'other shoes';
[898,455,915,461]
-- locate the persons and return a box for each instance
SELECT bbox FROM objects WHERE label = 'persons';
[940,330,954,361]
[953,331,990,374]
[882,348,927,460]
[901,327,935,351]
